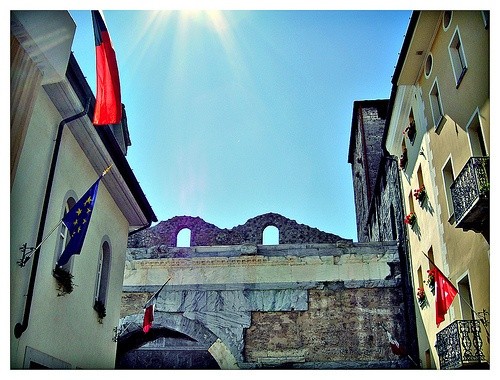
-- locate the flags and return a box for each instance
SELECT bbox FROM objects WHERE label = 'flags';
[91,10,122,125]
[56,178,100,265]
[431,265,457,327]
[143,292,158,334]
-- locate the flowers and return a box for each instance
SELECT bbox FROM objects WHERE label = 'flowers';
[423,270,435,287]
[413,185,425,201]
[404,212,417,224]
[416,287,425,301]
